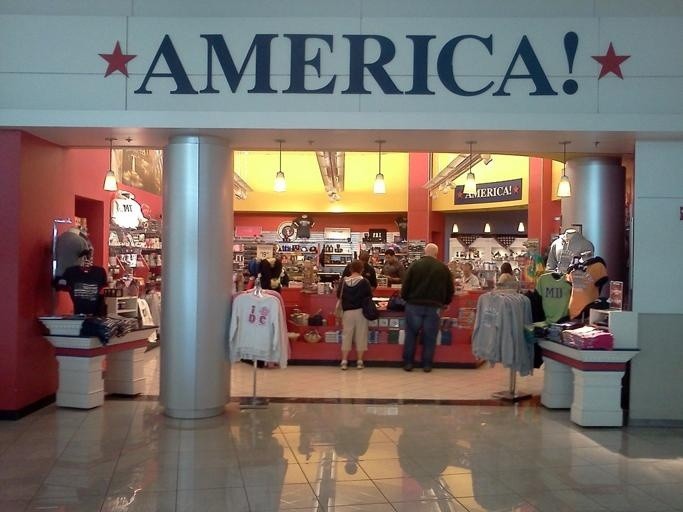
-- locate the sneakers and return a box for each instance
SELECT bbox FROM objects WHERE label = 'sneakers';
[404,367,412,370]
[357,363,364,369]
[340,363,348,370]
[424,366,431,372]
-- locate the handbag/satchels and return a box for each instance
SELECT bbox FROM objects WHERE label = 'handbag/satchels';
[387,290,405,312]
[334,299,343,319]
[362,298,379,320]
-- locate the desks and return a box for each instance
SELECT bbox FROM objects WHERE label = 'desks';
[267,288,489,368]
[538,338,641,427]
[37,315,159,410]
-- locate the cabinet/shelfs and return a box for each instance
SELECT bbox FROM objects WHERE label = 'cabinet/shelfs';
[106,296,139,329]
[590,308,638,350]
[108,231,161,292]
[232,240,408,281]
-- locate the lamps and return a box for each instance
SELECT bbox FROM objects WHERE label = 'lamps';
[103,138,118,191]
[235,188,246,200]
[557,141,571,197]
[274,140,287,192]
[439,141,493,194]
[374,140,386,194]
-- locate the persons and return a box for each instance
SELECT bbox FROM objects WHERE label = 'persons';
[401,242,455,372]
[55,227,90,276]
[79,227,94,267]
[460,263,479,290]
[500,262,513,276]
[380,249,404,288]
[369,248,380,264]
[566,250,609,321]
[337,259,373,370]
[341,250,377,291]
[545,228,594,274]
